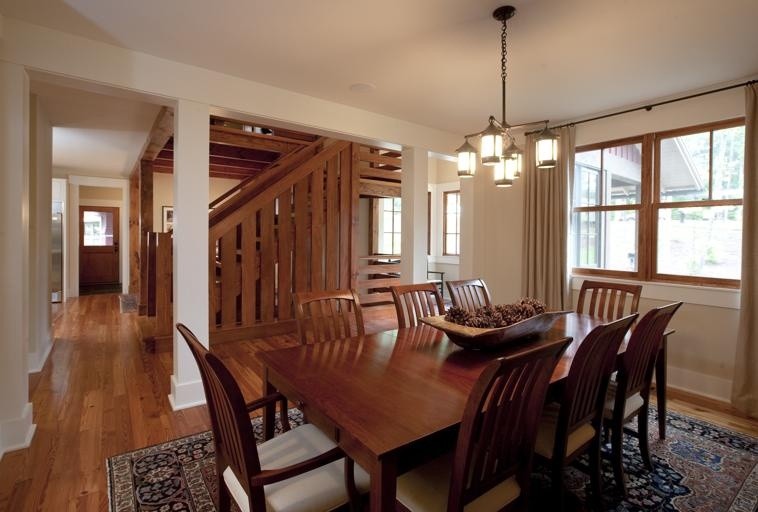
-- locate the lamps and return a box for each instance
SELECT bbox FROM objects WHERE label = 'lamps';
[452,3,562,187]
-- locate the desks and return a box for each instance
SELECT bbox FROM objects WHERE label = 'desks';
[250,311,677,511]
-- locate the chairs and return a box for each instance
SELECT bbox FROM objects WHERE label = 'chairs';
[289,288,369,345]
[527,313,638,501]
[602,301,683,500]
[447,277,491,304]
[394,340,573,510]
[177,324,371,509]
[575,279,645,314]
[389,281,446,328]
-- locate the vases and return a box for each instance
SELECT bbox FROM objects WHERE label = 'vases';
[419,311,575,356]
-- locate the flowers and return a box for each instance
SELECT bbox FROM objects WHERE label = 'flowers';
[448,297,555,327]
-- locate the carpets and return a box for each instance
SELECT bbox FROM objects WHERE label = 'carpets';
[102,386,758,510]
